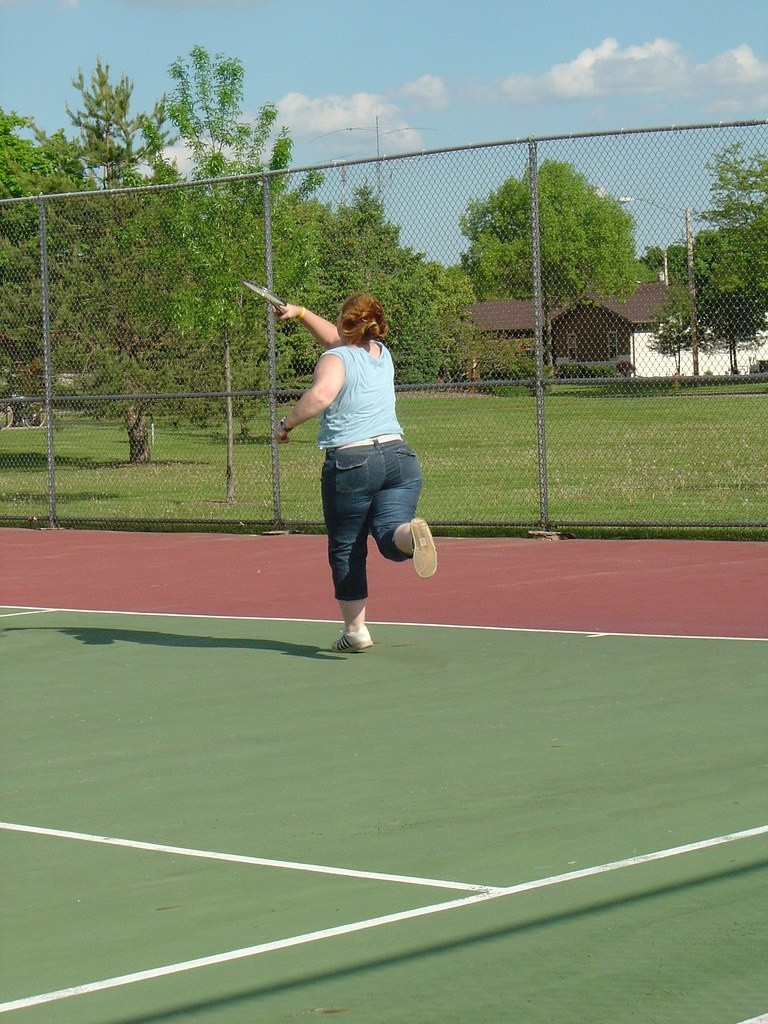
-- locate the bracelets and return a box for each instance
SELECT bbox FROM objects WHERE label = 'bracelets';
[298,307,306,321]
[280,417,294,431]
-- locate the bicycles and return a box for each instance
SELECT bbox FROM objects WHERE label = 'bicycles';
[0,394,47,433]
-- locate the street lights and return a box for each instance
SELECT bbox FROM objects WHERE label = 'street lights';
[619,196,699,377]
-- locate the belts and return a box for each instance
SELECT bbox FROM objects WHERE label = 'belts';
[327,433,403,452]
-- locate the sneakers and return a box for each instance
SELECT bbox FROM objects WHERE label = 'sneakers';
[410,517,438,578]
[330,625,374,653]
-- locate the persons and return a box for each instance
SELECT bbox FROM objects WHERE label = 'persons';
[272,293,438,653]
